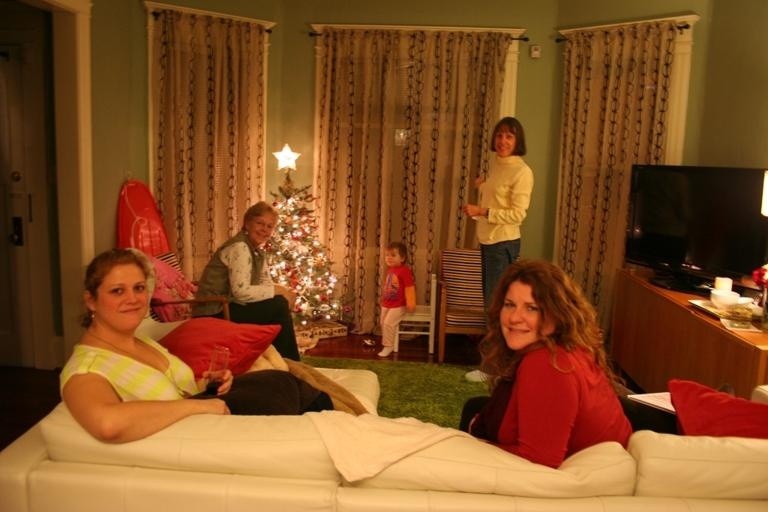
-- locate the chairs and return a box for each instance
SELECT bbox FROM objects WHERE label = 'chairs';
[393,274,437,353]
[438,248,520,361]
[151,250,230,322]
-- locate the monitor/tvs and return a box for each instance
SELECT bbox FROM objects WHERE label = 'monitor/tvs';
[625,165,768,296]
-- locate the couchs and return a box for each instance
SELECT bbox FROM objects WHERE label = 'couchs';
[1,368,768,512]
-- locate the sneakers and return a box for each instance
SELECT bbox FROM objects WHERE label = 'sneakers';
[465,370,489,382]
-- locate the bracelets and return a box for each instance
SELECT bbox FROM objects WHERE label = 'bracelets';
[485,207,490,219]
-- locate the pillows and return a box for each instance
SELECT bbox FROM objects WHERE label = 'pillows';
[152,254,199,322]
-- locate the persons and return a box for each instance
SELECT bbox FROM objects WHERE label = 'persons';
[59,248,235,445]
[376,243,416,357]
[459,258,634,466]
[461,117,534,382]
[191,202,302,364]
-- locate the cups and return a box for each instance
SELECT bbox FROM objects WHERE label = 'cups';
[714,276,733,292]
[709,288,740,312]
[203,345,231,397]
[729,304,753,329]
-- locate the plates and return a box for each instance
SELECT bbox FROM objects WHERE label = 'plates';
[688,298,764,319]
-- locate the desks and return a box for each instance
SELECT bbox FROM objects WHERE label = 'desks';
[609,268,768,402]
